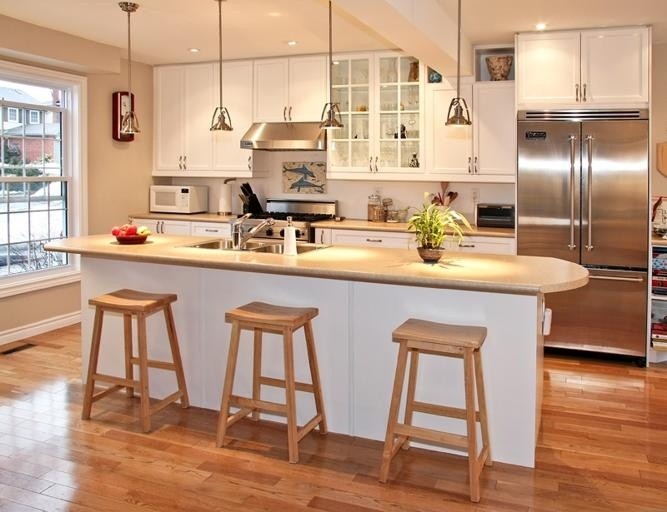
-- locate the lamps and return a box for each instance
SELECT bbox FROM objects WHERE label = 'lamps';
[316,1,345,131]
[206,1,236,133]
[445,0,472,129]
[116,0,142,137]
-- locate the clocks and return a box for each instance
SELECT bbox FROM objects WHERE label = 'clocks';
[111,87,135,142]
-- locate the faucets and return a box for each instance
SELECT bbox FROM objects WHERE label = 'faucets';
[233,212,276,251]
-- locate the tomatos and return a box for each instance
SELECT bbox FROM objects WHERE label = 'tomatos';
[112,224,137,236]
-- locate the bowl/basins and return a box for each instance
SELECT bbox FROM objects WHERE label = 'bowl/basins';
[114,233,147,244]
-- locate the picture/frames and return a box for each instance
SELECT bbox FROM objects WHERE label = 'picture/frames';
[473,44,516,82]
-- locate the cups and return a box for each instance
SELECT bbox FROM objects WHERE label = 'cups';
[397,211,407,223]
[484,56,512,81]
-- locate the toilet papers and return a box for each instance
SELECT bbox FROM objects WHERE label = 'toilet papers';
[284,227,296,255]
[218,184,232,212]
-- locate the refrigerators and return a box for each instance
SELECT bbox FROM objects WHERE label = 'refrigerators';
[514,111,648,363]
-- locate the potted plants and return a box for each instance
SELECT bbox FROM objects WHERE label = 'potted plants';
[402,192,472,263]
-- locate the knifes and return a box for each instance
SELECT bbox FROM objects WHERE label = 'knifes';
[237,182,252,206]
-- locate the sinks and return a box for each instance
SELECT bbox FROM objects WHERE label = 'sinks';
[184,239,266,255]
[253,241,331,256]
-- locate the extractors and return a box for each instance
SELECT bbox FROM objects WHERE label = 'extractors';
[239,122,327,152]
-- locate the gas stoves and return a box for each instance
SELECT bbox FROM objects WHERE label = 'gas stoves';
[237,211,333,240]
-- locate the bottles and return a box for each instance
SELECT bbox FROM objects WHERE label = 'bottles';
[408,154,418,167]
[367,194,397,223]
[429,71,440,83]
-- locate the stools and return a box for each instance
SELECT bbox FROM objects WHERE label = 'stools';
[376,318,493,503]
[81,286,189,433]
[211,300,330,462]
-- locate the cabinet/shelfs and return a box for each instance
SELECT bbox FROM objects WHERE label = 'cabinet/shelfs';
[151,61,214,178]
[510,19,653,115]
[323,44,428,183]
[211,54,268,180]
[132,218,193,237]
[645,191,666,370]
[423,79,516,188]
[251,51,330,128]
[192,220,231,238]
[314,229,415,250]
[410,233,517,259]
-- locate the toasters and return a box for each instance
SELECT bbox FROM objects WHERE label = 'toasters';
[475,203,514,229]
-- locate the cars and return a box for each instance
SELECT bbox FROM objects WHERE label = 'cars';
[0,181,64,265]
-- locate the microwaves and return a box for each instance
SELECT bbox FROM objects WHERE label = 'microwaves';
[149,184,210,214]
